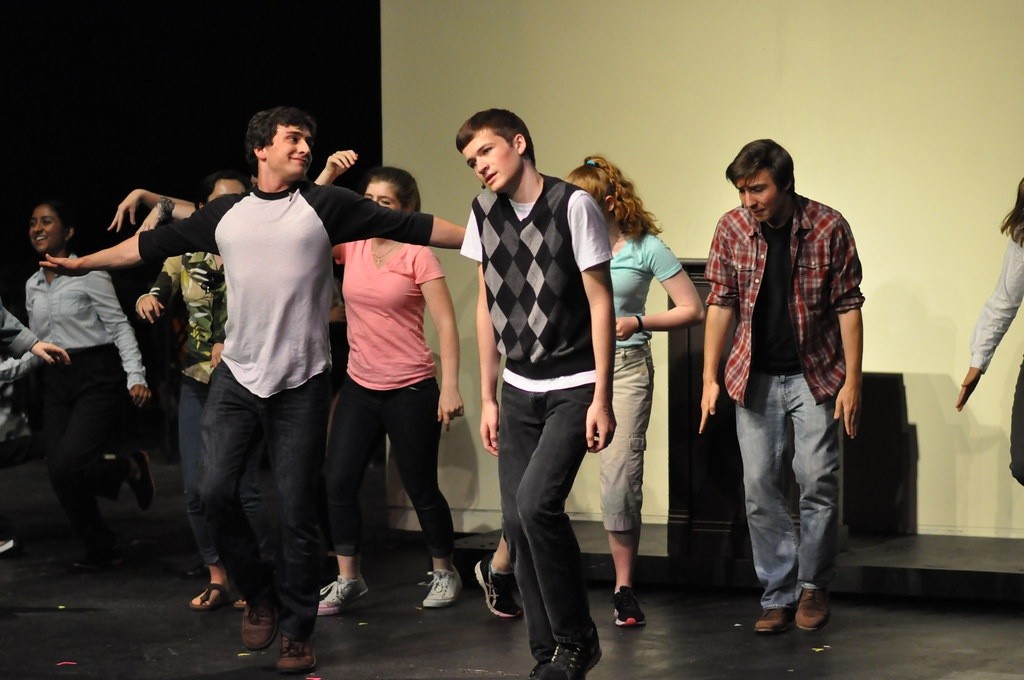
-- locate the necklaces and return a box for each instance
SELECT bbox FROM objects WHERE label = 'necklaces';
[372,242,401,265]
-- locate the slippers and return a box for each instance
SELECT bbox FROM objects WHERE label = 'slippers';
[188,585,230,610]
[233,598,247,611]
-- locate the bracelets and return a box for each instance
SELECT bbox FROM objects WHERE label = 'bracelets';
[635,316,643,334]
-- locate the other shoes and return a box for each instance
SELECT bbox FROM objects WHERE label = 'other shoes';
[0,534,22,558]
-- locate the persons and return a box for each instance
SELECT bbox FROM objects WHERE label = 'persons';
[0,106,466,672]
[456,108,617,680]
[699,140,866,632]
[475,155,705,626]
[956,178,1023,488]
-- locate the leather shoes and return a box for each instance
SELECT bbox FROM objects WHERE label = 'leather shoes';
[794,588,830,631]
[754,607,796,632]
[242,594,279,650]
[276,635,318,673]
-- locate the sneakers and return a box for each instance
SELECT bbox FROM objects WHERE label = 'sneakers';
[528,658,551,680]
[611,586,647,626]
[538,633,601,680]
[132,450,156,504]
[422,564,462,608]
[474,552,524,619]
[73,543,123,569]
[316,574,368,616]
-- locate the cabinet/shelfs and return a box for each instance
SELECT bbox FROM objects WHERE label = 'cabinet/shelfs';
[668,262,753,561]
